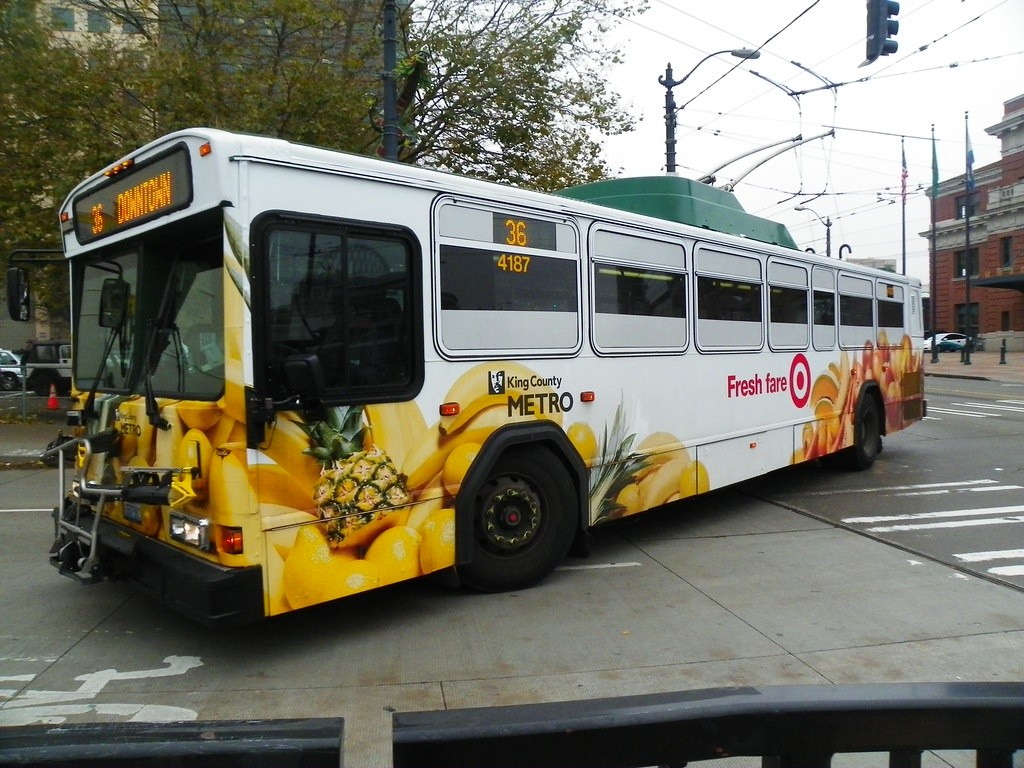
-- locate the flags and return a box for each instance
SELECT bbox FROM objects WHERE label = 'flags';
[901,150,909,204]
[931,142,940,196]
[967,133,976,190]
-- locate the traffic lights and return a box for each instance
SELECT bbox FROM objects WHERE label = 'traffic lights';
[866,1,900,58]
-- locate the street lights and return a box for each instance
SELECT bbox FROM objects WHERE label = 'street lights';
[658,47,761,172]
[794,206,831,257]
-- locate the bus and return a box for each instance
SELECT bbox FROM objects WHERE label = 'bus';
[43,129,926,616]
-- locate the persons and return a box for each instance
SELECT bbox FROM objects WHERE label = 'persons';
[320,286,389,369]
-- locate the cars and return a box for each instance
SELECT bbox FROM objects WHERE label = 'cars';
[0,350,24,392]
[923,333,970,352]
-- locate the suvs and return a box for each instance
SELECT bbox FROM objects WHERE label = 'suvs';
[20,340,127,398]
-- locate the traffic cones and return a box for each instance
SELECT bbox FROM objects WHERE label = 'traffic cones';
[46,383,59,410]
